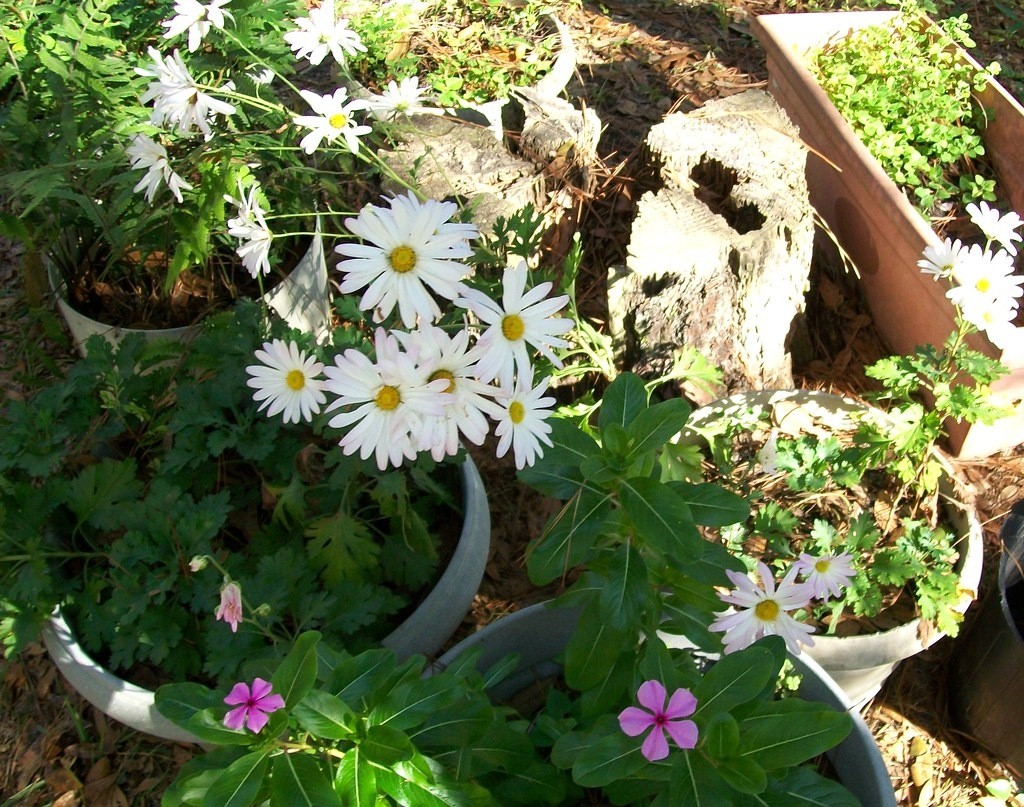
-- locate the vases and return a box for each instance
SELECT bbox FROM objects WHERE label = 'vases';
[425,587,897,807]
[25,423,493,754]
[948,496,1024,769]
[665,383,984,718]
[752,10,1024,462]
[43,188,334,398]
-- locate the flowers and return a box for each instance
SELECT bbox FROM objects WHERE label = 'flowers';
[128,0,1024,765]
